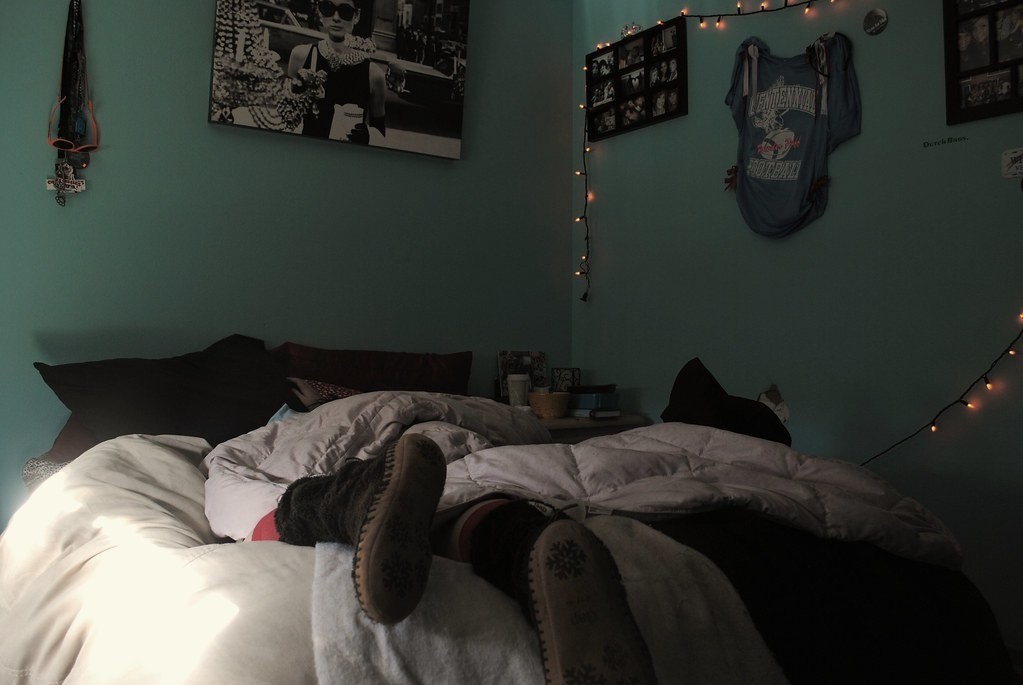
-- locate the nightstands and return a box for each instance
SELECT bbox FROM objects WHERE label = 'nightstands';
[540,417,649,444]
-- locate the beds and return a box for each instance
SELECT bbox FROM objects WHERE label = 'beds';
[0,390,1023,685]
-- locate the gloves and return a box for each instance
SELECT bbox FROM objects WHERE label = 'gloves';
[346,123,370,145]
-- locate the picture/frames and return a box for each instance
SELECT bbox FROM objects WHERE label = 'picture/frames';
[942,0,1023,128]
[497,350,548,397]
[585,16,688,143]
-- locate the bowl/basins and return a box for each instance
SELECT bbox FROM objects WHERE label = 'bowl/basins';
[527,392,568,418]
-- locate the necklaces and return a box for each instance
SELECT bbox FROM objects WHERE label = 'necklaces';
[318,38,365,72]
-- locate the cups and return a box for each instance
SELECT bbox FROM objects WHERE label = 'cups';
[507,374,530,408]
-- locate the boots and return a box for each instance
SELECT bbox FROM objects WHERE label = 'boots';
[274,435,446,622]
[469,504,655,685]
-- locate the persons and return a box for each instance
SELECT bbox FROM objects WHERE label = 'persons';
[651,31,677,55]
[652,87,679,118]
[595,108,615,133]
[591,81,615,107]
[592,60,612,76]
[620,95,646,125]
[395,23,443,67]
[202,376,658,685]
[287,0,386,146]
[619,46,641,70]
[952,0,1023,108]
[649,60,678,87]
[626,72,645,95]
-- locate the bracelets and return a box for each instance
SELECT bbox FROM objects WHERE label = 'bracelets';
[307,395,328,411]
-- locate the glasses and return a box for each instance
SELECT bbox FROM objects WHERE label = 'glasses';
[47,96,98,153]
[317,0,358,23]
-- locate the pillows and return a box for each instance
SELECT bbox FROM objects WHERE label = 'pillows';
[279,342,474,395]
[33,333,280,449]
[48,413,98,463]
